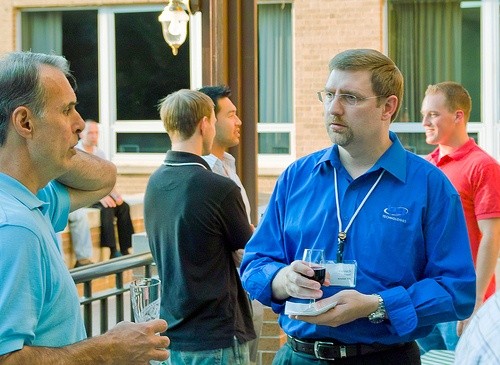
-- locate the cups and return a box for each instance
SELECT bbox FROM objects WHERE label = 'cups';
[131,278,161,323]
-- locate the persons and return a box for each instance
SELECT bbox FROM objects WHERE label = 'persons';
[238,49,476,365]
[142,89,258,364]
[53,205,98,270]
[414,82,500,355]
[453,289,500,365]
[0,49,171,364]
[74,119,135,260]
[197,84,264,365]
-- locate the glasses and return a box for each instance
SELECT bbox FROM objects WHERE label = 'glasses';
[317,87,392,106]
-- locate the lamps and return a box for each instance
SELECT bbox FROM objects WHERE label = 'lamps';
[157,0,193,57]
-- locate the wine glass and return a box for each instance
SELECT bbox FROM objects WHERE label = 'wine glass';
[301,248,326,312]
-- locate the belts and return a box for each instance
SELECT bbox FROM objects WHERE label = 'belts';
[286,334,413,361]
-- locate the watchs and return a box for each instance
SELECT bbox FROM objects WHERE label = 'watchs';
[368,293,387,325]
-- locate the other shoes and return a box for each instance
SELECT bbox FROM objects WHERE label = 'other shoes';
[74,259,93,269]
